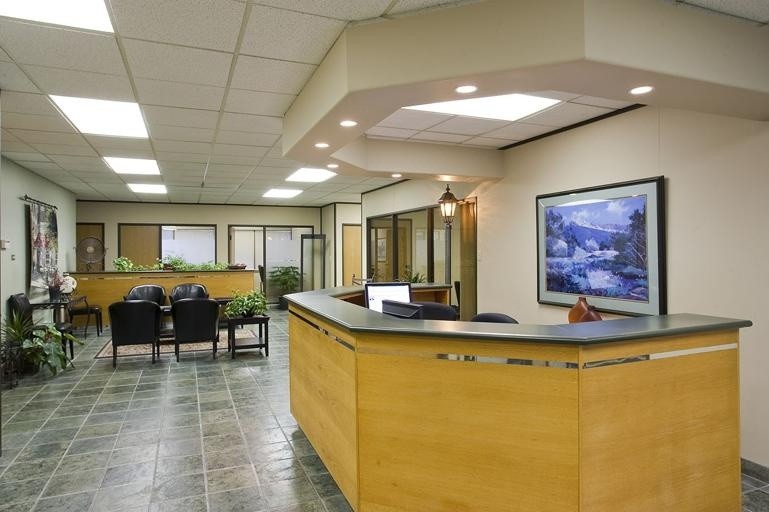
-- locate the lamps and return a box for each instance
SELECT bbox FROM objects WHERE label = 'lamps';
[437,183,458,285]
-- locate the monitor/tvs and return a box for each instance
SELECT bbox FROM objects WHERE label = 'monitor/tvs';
[382,299,425,320]
[364,281,413,313]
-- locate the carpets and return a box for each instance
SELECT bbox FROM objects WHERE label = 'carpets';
[93,329,263,359]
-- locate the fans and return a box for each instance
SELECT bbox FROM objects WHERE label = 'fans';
[76,237,105,272]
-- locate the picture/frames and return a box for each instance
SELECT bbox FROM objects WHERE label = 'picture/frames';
[536,176,667,317]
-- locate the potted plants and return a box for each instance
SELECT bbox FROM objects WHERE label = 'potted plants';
[224,288,269,319]
[0,308,85,389]
[266,266,302,310]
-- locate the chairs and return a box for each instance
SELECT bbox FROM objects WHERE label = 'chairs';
[123,285,166,329]
[108,300,166,368]
[411,302,460,361]
[63,294,103,336]
[7,293,74,364]
[464,313,532,365]
[169,283,209,305]
[171,298,222,362]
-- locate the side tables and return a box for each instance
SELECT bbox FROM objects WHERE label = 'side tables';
[224,311,271,359]
